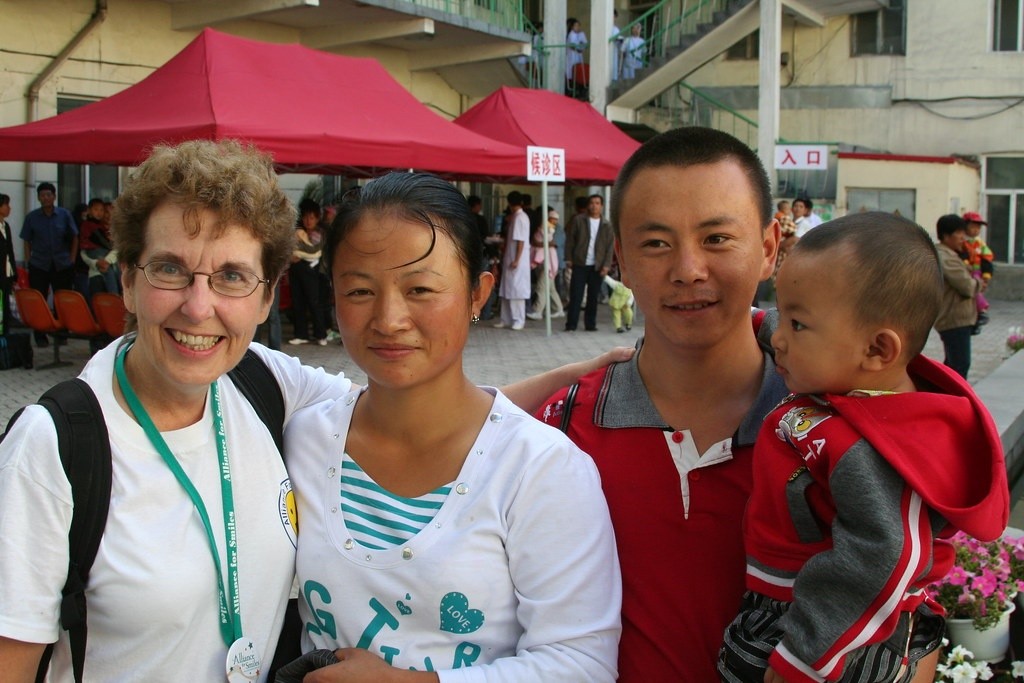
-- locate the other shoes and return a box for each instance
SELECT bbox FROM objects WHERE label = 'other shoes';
[617,328,624,333]
[564,326,575,332]
[493,320,511,328]
[971,324,980,335]
[317,338,327,346]
[552,310,565,318]
[512,320,524,329]
[976,312,989,325]
[33,331,48,347]
[289,337,310,346]
[626,323,631,331]
[585,327,598,331]
[526,311,543,321]
[57,336,67,345]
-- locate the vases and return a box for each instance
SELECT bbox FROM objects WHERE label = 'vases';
[944,600,1016,665]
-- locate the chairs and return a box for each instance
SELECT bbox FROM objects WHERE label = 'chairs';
[13,287,129,370]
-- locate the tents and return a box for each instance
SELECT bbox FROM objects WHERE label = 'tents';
[444,85,641,185]
[0,27,550,334]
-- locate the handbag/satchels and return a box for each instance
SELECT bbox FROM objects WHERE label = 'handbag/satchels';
[0,332,33,370]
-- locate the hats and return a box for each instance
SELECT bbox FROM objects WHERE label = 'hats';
[963,211,988,226]
[549,211,559,220]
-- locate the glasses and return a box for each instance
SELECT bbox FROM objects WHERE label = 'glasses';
[130,261,271,299]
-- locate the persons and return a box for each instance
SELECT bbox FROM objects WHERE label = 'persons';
[620,22,648,80]
[0,182,122,353]
[612,9,620,82]
[775,197,822,242]
[566,19,587,80]
[254,201,339,351]
[603,274,634,331]
[286,174,621,683]
[518,23,544,70]
[960,213,995,332]
[468,191,608,330]
[565,195,614,331]
[935,215,976,376]
[536,125,946,682]
[0,138,635,683]
[714,210,1007,682]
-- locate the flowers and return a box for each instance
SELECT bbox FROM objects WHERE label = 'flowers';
[927,527,1024,632]
[934,644,994,683]
